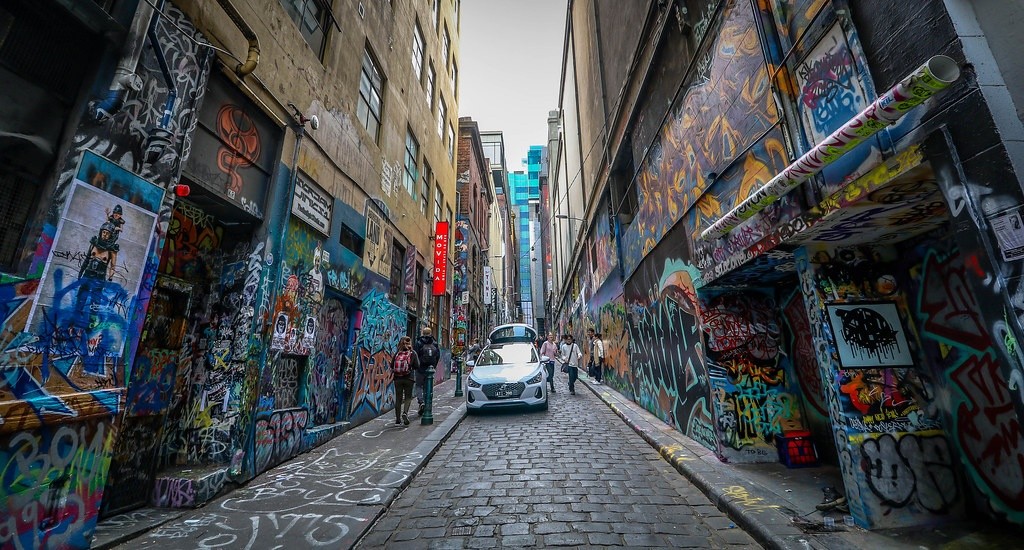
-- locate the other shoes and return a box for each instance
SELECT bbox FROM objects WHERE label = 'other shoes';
[418,403,425,416]
[571,391,575,395]
[395,422,402,426]
[591,379,602,385]
[552,389,556,392]
[402,412,410,425]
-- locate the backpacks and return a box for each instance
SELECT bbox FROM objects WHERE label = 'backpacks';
[394,350,413,377]
[419,338,438,366]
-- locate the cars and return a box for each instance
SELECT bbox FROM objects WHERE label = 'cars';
[464,325,552,412]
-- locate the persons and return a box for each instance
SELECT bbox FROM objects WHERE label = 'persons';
[415,326,440,415]
[1009,215,1020,231]
[535,332,560,393]
[390,336,421,427]
[468,338,482,364]
[561,334,582,395]
[588,328,605,384]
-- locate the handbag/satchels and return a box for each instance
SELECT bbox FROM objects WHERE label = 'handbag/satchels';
[590,366,596,377]
[561,362,569,373]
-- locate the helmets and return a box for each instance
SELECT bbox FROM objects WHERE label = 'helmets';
[100,222,115,233]
[113,204,123,215]
